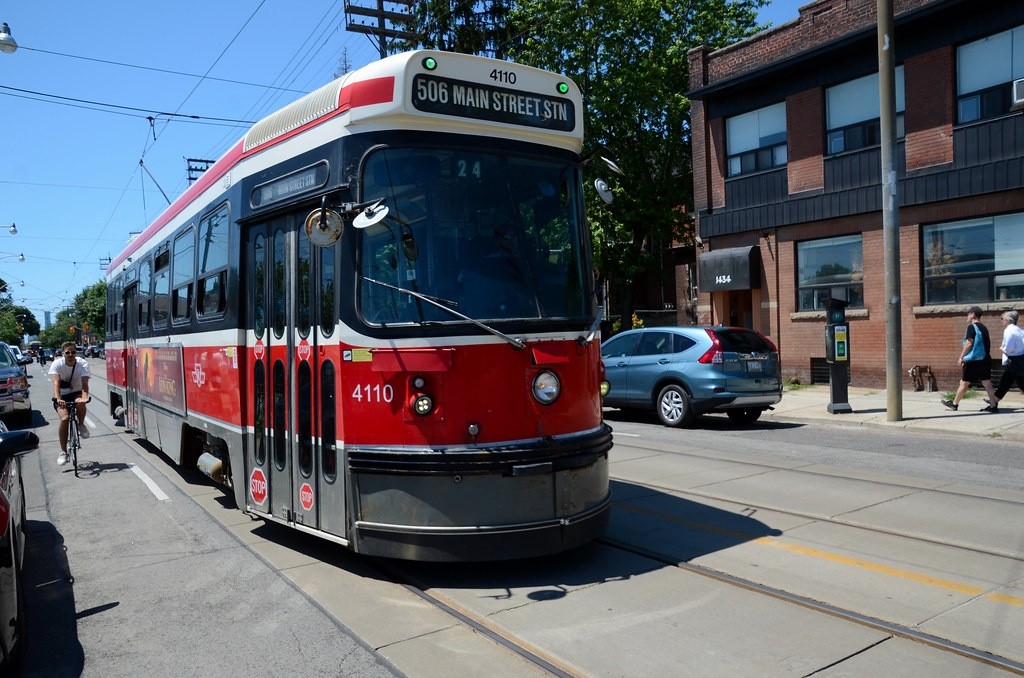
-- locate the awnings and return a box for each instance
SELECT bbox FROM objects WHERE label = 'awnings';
[698,245,761,293]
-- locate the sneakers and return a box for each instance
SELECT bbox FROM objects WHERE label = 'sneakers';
[78,424,89,439]
[57,451,67,466]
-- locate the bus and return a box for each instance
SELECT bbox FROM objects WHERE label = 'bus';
[27,341,42,357]
[100,50,618,564]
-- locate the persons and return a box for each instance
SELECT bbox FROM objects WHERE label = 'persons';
[48,342,91,465]
[983,311,1024,407]
[38,347,47,366]
[941,307,998,414]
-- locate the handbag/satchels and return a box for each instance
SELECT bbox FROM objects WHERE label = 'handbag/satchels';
[59,380,72,389]
[961,324,986,361]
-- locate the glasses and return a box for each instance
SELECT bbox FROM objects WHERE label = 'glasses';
[64,351,76,355]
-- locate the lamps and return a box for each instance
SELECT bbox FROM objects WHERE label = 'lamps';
[0,23,18,55]
[8,222,18,235]
[18,253,26,262]
[20,281,24,287]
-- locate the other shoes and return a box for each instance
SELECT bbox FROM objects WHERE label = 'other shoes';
[979,406,1000,414]
[942,400,957,411]
[983,398,999,406]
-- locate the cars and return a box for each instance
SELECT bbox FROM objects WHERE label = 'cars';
[604,323,784,429]
[0,341,33,428]
[1,420,40,678]
[51,396,92,477]
[36,345,106,363]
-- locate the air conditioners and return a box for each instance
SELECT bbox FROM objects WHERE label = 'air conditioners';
[1012,79,1024,106]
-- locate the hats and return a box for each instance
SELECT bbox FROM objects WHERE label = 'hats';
[968,307,983,316]
